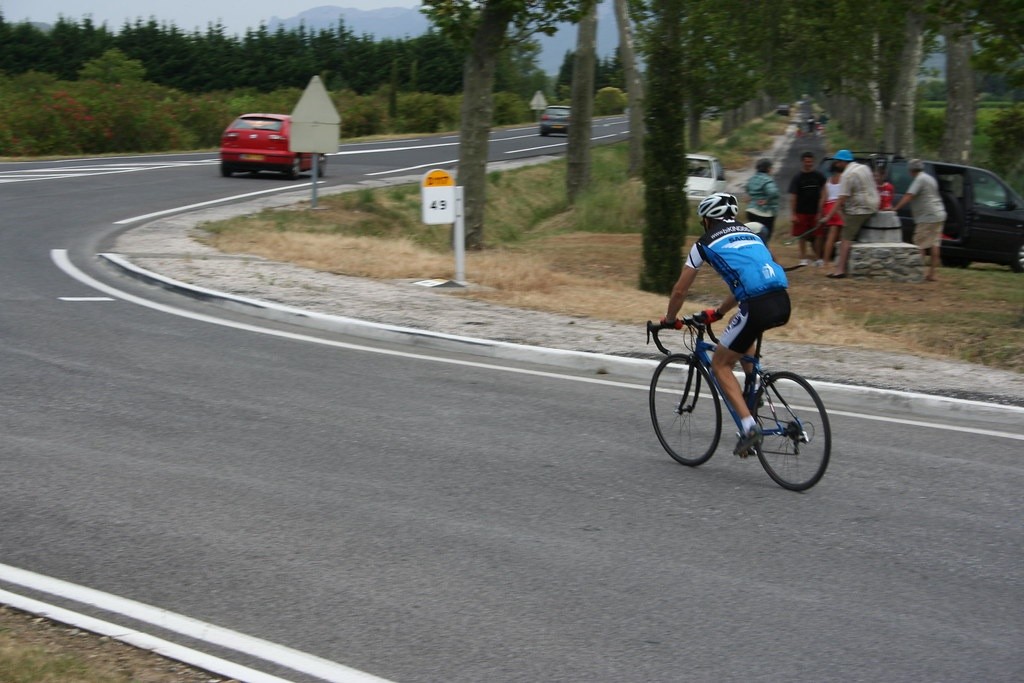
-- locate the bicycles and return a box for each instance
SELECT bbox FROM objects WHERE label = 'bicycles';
[644,311,832,493]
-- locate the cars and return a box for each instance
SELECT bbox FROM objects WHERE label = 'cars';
[681,153,727,207]
[540,104,573,136]
[774,104,791,117]
[220,112,328,180]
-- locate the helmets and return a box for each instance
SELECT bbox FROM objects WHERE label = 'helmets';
[696,192,739,221]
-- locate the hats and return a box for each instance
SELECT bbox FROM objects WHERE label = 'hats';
[828,149,855,161]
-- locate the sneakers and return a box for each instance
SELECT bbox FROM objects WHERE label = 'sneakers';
[745,392,764,409]
[732,424,762,457]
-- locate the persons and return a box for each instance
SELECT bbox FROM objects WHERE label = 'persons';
[744,158,778,246]
[875,166,893,211]
[882,159,946,281]
[660,193,792,455]
[820,150,879,278]
[788,152,827,259]
[821,161,845,265]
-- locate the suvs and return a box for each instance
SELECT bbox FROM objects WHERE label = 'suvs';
[816,150,1024,274]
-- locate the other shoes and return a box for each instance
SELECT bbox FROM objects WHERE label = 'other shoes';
[814,259,823,266]
[799,257,809,266]
[824,272,846,279]
